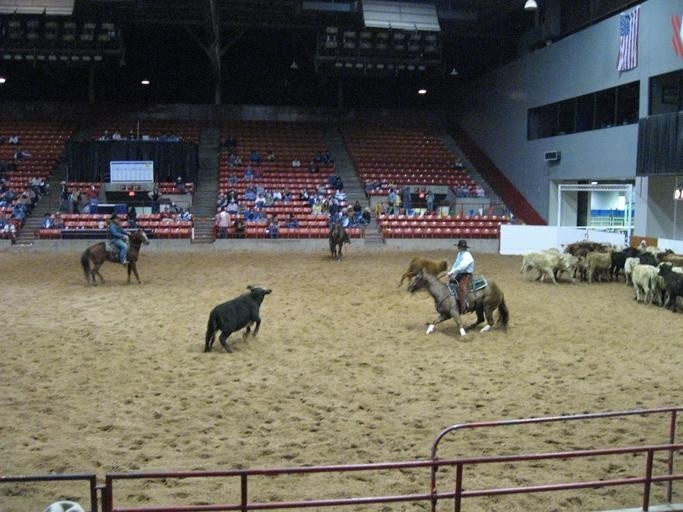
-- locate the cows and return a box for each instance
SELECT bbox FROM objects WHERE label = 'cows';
[395,256,448,289]
[204,285,273,353]
[518,238,683,313]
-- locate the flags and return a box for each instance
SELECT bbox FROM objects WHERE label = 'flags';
[615,5,640,71]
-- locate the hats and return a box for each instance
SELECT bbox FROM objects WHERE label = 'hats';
[110,213,119,220]
[455,240,469,248]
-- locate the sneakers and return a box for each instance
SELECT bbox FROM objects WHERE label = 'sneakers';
[123,261,129,265]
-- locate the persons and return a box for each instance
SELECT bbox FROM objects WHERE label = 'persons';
[453,157,464,171]
[97,130,186,143]
[0,133,51,243]
[210,130,371,240]
[445,239,475,313]
[363,179,516,225]
[105,214,132,265]
[39,176,195,238]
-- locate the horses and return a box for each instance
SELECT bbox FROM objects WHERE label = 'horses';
[328,219,345,262]
[407,267,509,337]
[81,227,150,287]
